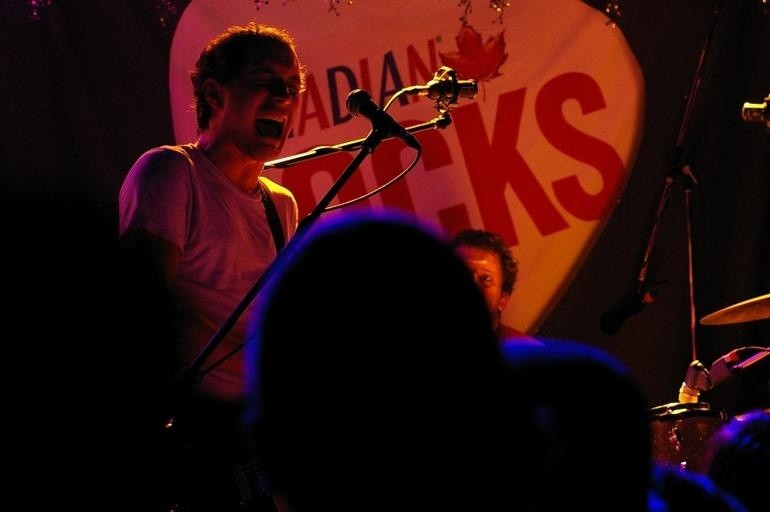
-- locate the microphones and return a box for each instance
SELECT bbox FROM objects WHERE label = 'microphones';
[404,64,479,113]
[678,359,711,404]
[345,88,421,151]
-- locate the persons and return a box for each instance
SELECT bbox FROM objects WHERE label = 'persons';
[3,207,254,510]
[649,408,767,508]
[448,229,535,339]
[262,218,509,510]
[116,24,307,402]
[495,340,649,510]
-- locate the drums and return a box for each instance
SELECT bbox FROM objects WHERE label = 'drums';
[652,402,728,477]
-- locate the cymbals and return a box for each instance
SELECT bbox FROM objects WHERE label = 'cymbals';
[700,294,770,326]
